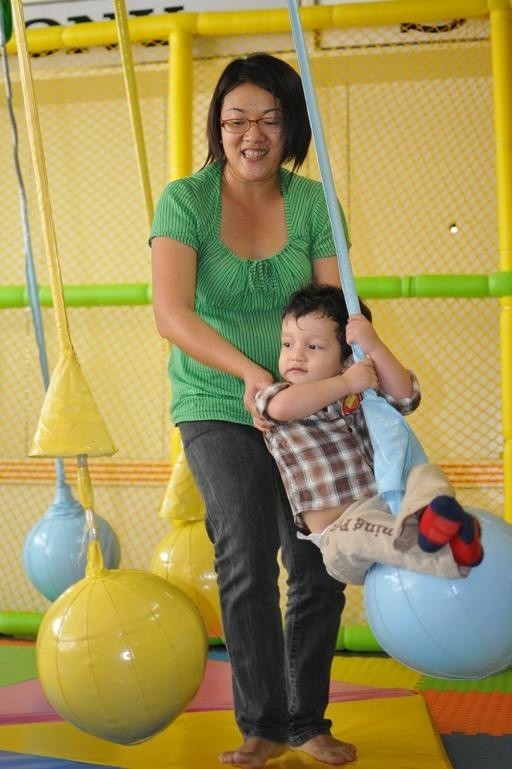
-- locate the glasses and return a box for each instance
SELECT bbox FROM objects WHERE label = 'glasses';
[219,117,285,133]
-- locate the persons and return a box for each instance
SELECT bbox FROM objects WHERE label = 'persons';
[249,282,487,589]
[143,49,361,767]
